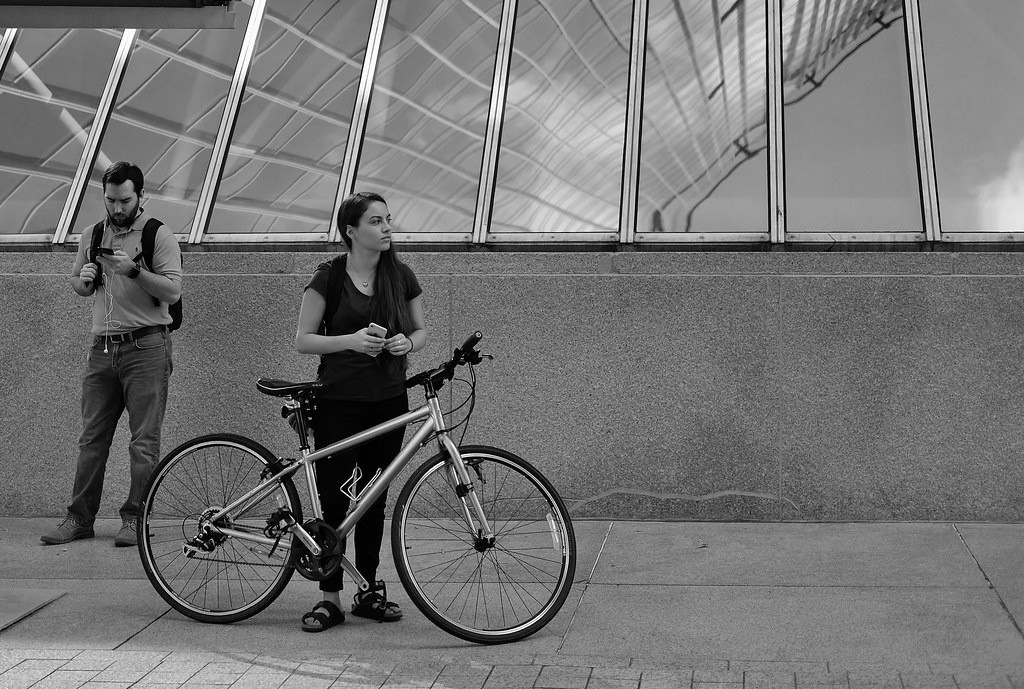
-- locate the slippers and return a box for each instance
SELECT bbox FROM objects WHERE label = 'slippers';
[302,601,345,631]
[351,593,403,620]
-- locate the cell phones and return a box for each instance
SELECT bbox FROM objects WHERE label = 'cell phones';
[97,247,115,259]
[366,323,387,358]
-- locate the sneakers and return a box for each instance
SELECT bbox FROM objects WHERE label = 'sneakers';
[115,518,138,546]
[41,517,95,543]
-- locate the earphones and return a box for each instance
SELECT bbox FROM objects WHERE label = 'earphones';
[104,345,108,353]
[102,273,107,277]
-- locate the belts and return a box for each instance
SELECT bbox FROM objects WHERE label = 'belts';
[101,324,164,343]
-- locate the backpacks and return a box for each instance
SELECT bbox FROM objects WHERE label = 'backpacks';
[91,218,184,331]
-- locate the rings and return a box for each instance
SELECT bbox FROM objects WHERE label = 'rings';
[398,340,400,344]
[370,348,372,351]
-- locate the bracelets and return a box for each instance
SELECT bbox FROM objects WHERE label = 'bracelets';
[405,336,413,354]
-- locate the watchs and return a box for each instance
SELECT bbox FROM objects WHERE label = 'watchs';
[127,264,141,279]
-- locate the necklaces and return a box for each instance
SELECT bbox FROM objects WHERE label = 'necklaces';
[348,252,368,287]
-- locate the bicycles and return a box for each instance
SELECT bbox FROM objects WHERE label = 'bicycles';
[136,331,577,644]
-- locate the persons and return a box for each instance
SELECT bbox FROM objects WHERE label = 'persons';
[39,160,184,546]
[296,190,426,632]
